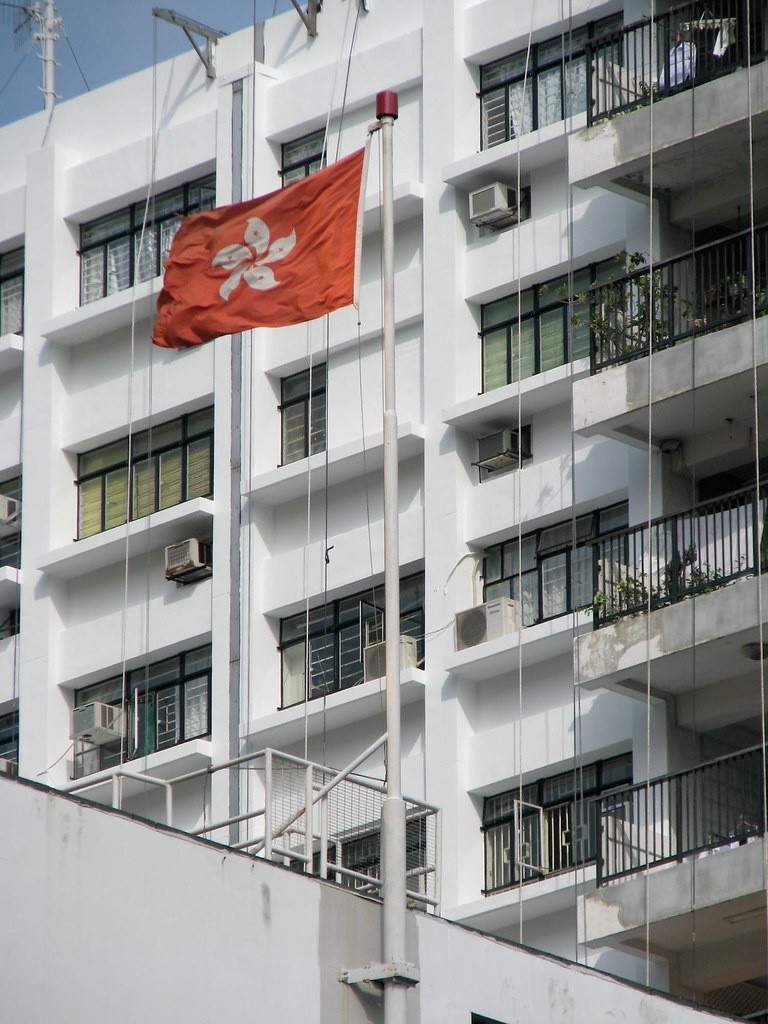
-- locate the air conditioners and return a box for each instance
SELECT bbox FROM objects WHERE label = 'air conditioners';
[455,597,522,652]
[165,538,212,586]
[0,493,20,539]
[471,430,532,472]
[69,701,128,746]
[364,634,417,685]
[469,181,527,231]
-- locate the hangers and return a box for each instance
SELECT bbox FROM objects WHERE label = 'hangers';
[727,813,760,839]
[678,4,736,32]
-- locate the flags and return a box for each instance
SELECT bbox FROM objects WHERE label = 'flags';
[151,134,372,349]
